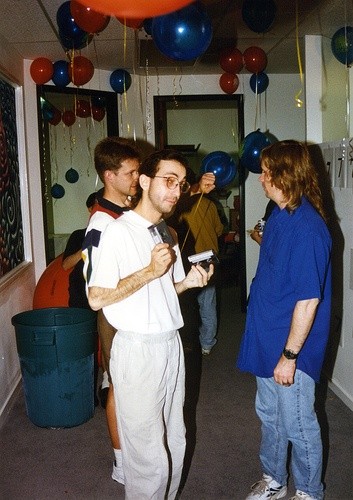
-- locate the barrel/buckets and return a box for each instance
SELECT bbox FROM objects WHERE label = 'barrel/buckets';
[11,307,97,428]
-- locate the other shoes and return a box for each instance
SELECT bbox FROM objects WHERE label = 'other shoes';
[99,385,109,409]
[201,337,217,356]
[111,460,124,486]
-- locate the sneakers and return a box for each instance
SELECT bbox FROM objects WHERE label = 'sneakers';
[288,489,317,500]
[245,472,288,500]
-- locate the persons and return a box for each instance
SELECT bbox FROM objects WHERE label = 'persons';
[62,136,226,500]
[235,140,335,500]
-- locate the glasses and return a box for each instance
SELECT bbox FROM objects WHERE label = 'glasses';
[145,174,191,194]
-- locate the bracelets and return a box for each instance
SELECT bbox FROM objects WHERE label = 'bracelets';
[284,348,298,360]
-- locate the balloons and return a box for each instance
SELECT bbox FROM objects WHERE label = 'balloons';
[43,102,61,125]
[71,0,279,95]
[331,26,353,66]
[91,94,108,121]
[30,57,53,85]
[241,129,272,175]
[51,60,71,87]
[56,0,95,50]
[62,110,76,127]
[68,56,94,86]
[65,169,79,183]
[73,100,91,118]
[109,69,132,95]
[201,151,236,187]
[51,183,65,199]
